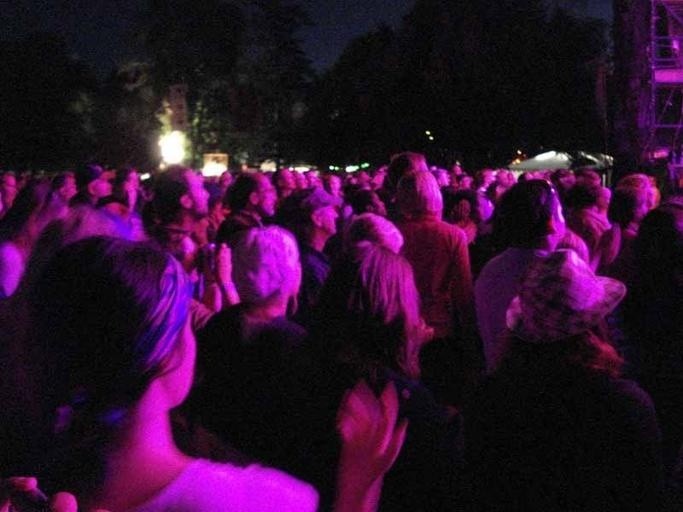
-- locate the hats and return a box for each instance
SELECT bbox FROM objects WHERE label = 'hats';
[506,248,625,343]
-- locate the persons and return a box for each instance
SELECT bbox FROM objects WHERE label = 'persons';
[1,150,681,511]
